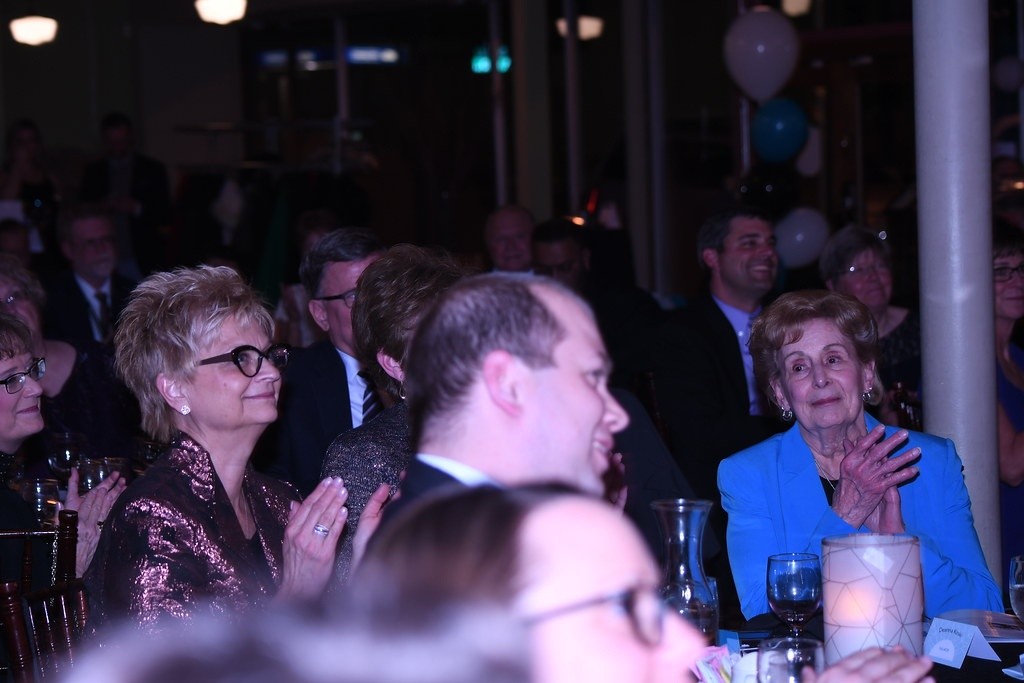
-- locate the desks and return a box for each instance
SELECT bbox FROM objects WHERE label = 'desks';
[727,610,1024,683]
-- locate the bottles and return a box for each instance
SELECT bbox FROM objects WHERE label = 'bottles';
[649,498,719,647]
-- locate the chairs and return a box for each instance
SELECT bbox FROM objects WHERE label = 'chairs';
[0,509,94,683]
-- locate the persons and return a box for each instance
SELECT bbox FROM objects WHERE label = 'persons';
[0,170,1024,683]
[71,113,169,212]
[0,119,59,225]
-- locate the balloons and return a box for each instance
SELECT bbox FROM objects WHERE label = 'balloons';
[723,11,800,100]
[752,101,807,161]
[773,207,829,270]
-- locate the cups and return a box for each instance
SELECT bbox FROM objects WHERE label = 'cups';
[706,577,719,612]
[756,638,825,683]
[1008,555,1024,629]
[18,430,170,530]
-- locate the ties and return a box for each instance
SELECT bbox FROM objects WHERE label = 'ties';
[748,337,772,414]
[358,369,379,425]
[95,291,110,341]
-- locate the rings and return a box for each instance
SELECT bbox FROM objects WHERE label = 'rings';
[96,521,103,529]
[314,524,329,536]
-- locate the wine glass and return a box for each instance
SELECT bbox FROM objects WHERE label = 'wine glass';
[765,552,822,666]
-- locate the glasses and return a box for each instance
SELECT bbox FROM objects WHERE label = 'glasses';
[0,356,47,394]
[834,258,891,278]
[195,344,289,377]
[315,287,358,309]
[525,582,664,646]
[992,260,1024,282]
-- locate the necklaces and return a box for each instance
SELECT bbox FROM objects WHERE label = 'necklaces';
[815,459,835,491]
[242,489,249,538]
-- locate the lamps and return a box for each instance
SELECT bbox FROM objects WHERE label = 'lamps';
[820,532,921,667]
